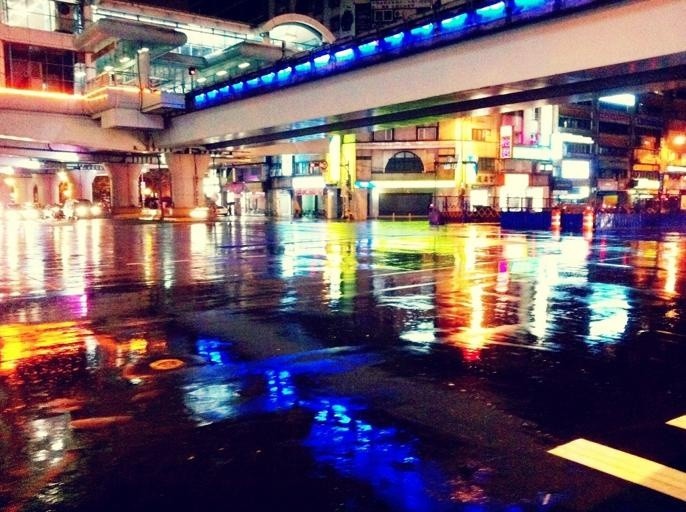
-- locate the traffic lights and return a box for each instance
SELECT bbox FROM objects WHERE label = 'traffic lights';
[188,67,195,75]
